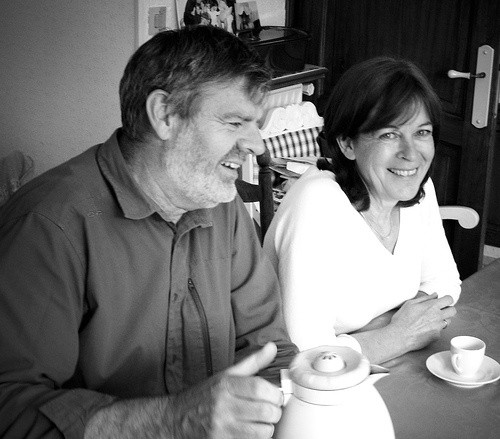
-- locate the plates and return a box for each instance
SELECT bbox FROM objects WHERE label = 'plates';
[426,350,500,388]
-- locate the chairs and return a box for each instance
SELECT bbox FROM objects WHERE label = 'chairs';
[236,102,479,245]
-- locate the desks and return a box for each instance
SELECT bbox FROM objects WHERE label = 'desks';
[354,254,500,439]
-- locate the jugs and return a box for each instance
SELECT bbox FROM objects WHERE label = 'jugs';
[253,347,395,439]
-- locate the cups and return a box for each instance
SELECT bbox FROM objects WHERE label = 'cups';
[451,336,486,376]
